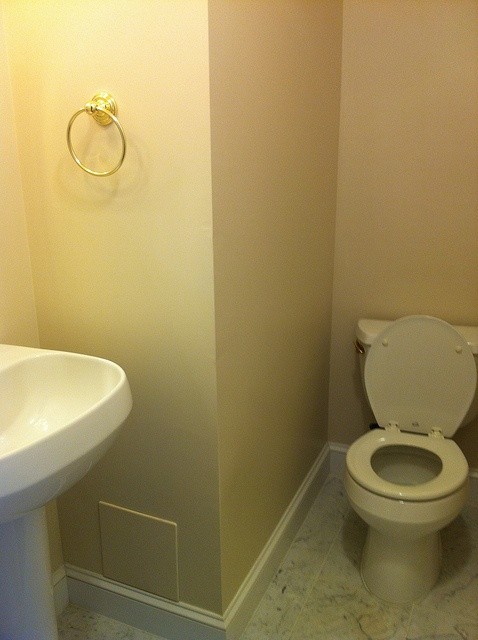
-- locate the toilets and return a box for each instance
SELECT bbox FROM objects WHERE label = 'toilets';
[343,315,477,603]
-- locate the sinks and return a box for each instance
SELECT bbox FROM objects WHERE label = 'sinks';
[0,342,133,526]
